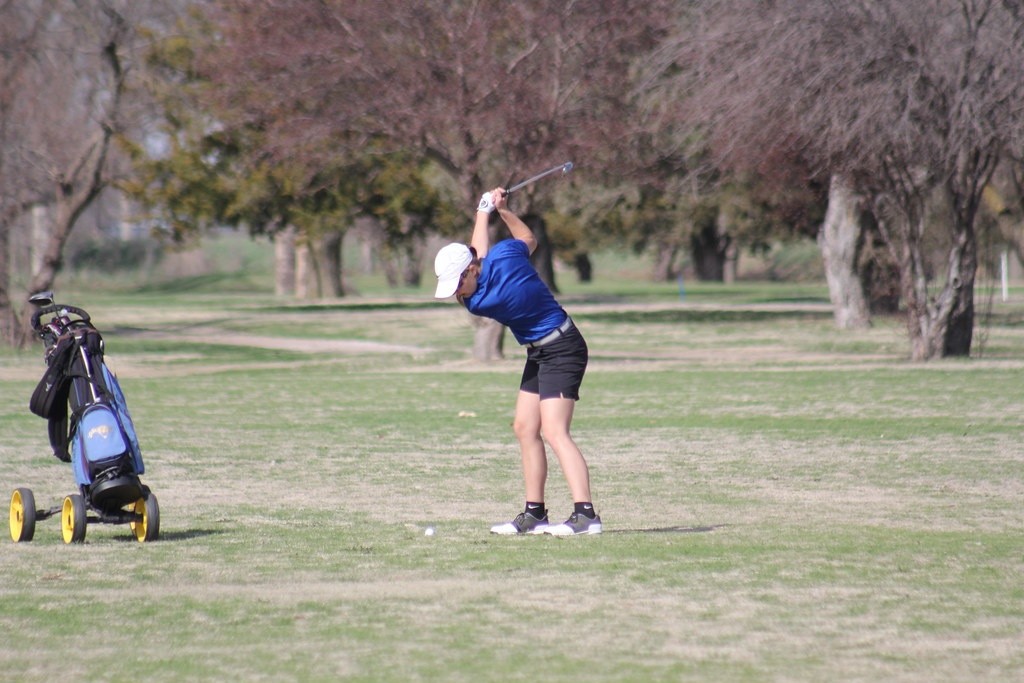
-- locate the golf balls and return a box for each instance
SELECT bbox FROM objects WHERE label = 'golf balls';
[425,527,434,535]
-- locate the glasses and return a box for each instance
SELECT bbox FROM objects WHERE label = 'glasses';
[458,268,469,288]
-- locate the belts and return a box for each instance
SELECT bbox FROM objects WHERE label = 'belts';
[525,317,572,348]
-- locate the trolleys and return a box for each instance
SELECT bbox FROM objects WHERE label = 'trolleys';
[9,304,160,543]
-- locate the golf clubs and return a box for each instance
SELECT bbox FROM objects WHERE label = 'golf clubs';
[501,162,573,197]
[29,291,74,337]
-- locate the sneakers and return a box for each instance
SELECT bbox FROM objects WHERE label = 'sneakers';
[491,509,549,535]
[543,511,602,536]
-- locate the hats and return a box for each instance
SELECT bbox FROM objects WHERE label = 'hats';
[434,243,474,298]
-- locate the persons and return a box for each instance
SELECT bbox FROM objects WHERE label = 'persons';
[434,187,603,538]
